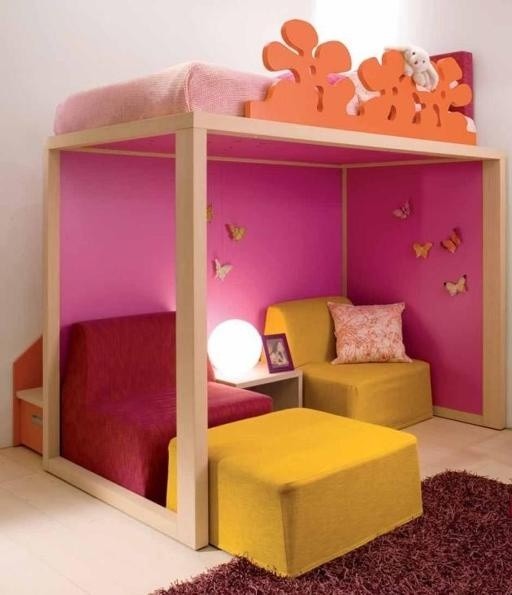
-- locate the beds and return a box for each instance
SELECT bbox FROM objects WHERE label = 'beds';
[43,20,508,553]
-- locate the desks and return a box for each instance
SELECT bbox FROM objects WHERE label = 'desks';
[214,366,305,408]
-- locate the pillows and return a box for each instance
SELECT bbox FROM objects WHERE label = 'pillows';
[326,300,413,364]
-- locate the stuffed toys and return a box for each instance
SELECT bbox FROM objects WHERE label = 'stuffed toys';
[386,42,441,92]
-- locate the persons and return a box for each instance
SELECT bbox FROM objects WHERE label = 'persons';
[271,342,288,367]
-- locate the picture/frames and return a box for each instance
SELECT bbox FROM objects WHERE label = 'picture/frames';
[263,333,295,374]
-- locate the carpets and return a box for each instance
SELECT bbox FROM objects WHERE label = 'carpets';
[152,470,511,594]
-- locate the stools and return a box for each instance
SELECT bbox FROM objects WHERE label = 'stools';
[163,407,425,578]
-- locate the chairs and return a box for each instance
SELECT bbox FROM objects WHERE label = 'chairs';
[60,311,272,505]
[261,295,433,430]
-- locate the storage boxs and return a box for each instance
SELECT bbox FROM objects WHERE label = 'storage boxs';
[19,400,43,455]
